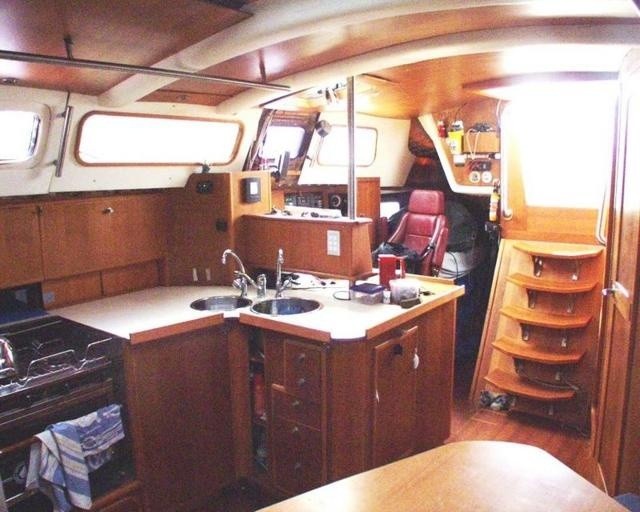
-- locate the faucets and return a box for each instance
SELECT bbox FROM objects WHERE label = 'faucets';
[232,269,267,297]
[221,248,249,297]
[273,247,289,298]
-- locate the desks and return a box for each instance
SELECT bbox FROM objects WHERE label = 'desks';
[252,440,636,511]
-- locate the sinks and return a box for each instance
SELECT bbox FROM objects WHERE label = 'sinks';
[249,296,324,317]
[189,295,253,312]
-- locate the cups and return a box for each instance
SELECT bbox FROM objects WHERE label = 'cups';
[376,253,407,291]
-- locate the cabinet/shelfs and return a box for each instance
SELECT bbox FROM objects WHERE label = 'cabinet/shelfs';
[227,313,374,501]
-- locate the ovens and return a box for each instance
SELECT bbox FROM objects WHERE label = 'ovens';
[0,364,135,512]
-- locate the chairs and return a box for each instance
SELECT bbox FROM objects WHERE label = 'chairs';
[370,189,449,277]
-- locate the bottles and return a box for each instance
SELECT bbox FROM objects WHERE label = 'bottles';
[251,372,264,420]
[436,119,445,137]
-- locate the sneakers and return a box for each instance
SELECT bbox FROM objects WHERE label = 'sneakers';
[480,389,510,411]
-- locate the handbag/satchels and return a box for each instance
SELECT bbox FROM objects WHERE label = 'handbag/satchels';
[375,241,417,273]
[445,224,476,249]
